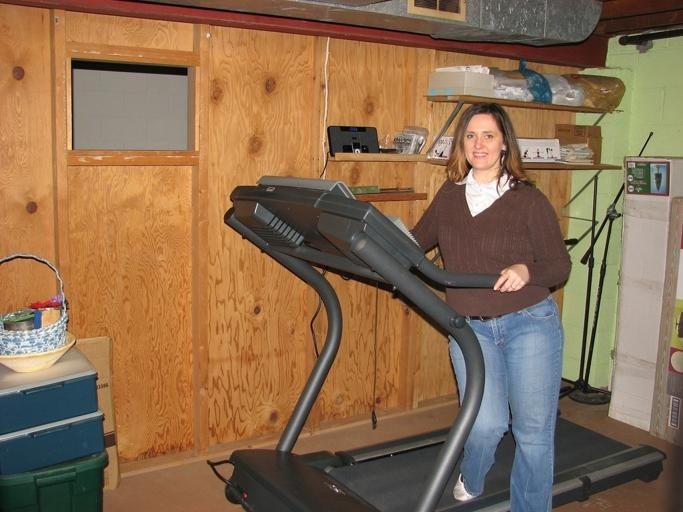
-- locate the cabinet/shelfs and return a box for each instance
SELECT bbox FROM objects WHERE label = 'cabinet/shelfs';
[327,149,429,205]
[424,92,624,172]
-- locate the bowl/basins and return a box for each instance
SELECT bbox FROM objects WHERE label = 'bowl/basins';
[0,332,77,374]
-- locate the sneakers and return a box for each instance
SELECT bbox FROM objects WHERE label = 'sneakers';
[450,472,479,501]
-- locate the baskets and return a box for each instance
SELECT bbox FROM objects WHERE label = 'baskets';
[0,254,67,355]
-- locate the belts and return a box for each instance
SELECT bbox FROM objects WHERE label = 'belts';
[459,313,506,323]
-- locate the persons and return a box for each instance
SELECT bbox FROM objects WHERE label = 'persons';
[394,101,573,512]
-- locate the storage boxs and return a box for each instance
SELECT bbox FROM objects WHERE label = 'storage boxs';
[0,451,111,512]
[0,409,107,472]
[0,348,100,438]
[554,122,604,163]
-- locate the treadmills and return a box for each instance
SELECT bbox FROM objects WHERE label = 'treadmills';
[223,177,666,512]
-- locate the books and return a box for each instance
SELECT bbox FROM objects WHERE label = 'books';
[558,145,595,166]
